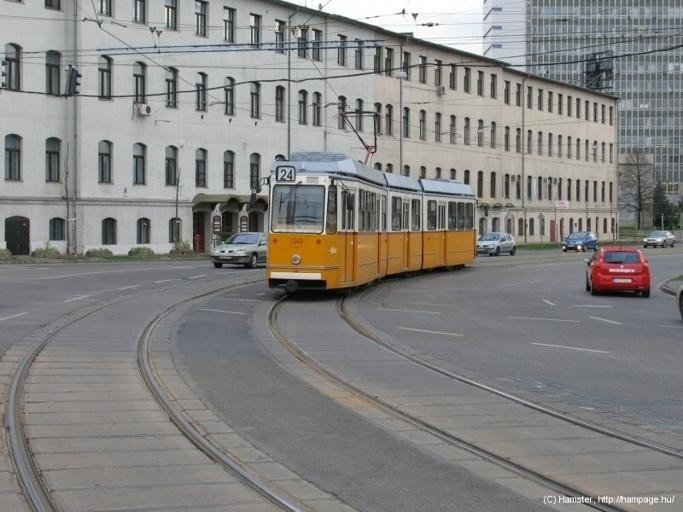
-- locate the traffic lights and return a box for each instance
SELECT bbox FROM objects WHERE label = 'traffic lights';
[63,63,81,99]
[0,57,7,90]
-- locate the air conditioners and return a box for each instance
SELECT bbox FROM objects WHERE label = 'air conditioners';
[136,103,152,116]
[510,174,518,181]
[438,85,446,95]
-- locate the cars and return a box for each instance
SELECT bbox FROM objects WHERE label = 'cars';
[213,230,266,269]
[584,246,651,299]
[675,284,682,319]
[475,230,518,258]
[561,231,599,253]
[643,230,676,249]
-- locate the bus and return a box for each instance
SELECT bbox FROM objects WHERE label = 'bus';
[249,151,477,297]
